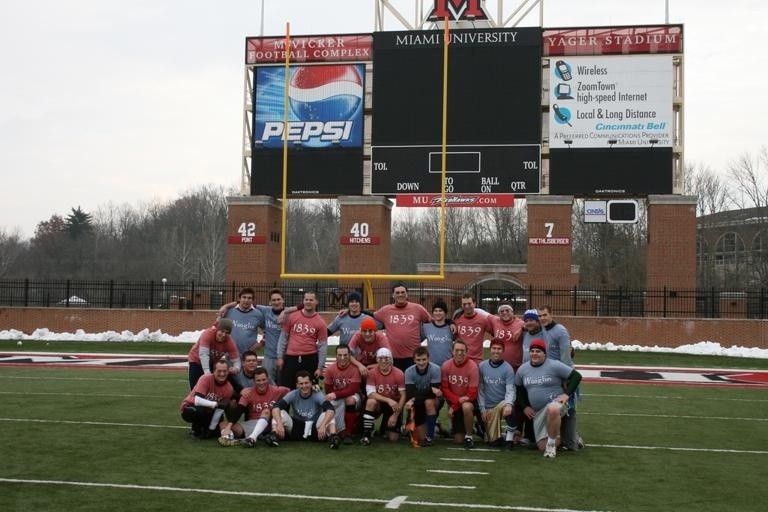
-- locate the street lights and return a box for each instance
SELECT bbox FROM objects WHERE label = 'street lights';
[161,277,167,304]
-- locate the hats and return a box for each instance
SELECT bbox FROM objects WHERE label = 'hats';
[219,319,232,334]
[498,300,514,311]
[349,293,360,302]
[360,318,377,331]
[523,309,538,320]
[530,340,545,354]
[432,300,447,313]
[377,348,392,357]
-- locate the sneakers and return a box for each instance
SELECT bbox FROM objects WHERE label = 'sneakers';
[330,434,341,448]
[217,434,279,447]
[543,435,583,458]
[505,441,512,451]
[423,419,449,446]
[465,437,473,447]
[360,433,371,445]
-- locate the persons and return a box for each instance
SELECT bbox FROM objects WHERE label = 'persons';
[179,284,585,458]
